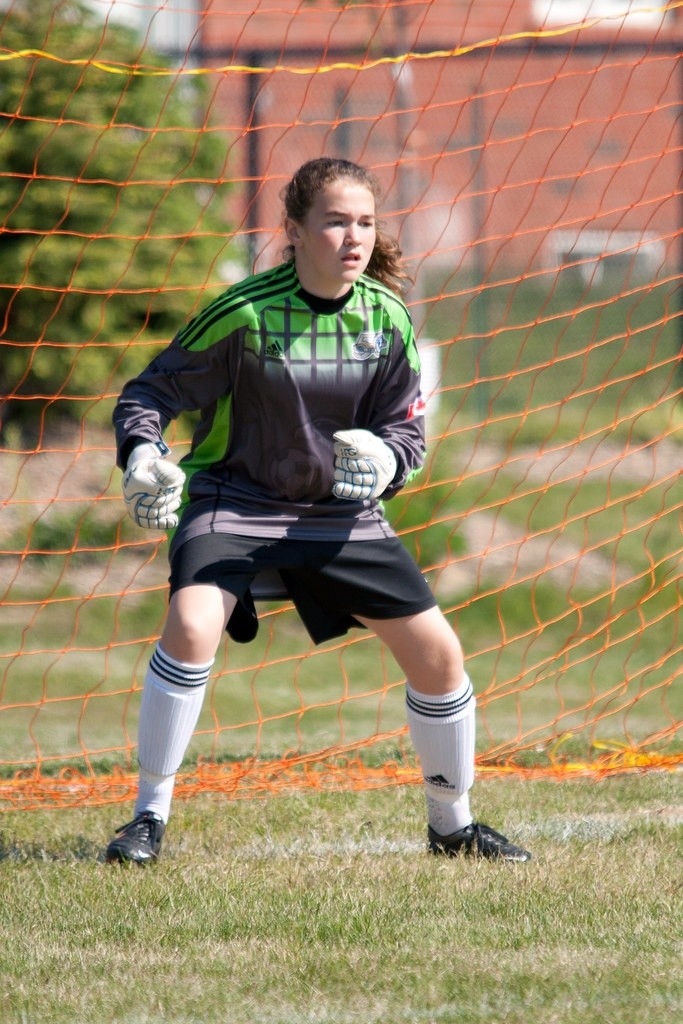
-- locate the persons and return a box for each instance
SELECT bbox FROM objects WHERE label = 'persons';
[103,157,529,869]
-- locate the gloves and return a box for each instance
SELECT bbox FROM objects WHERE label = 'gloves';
[334,429,397,501]
[122,440,186,530]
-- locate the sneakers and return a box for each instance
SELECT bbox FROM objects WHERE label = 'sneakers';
[427,821,532,861]
[107,812,167,864]
